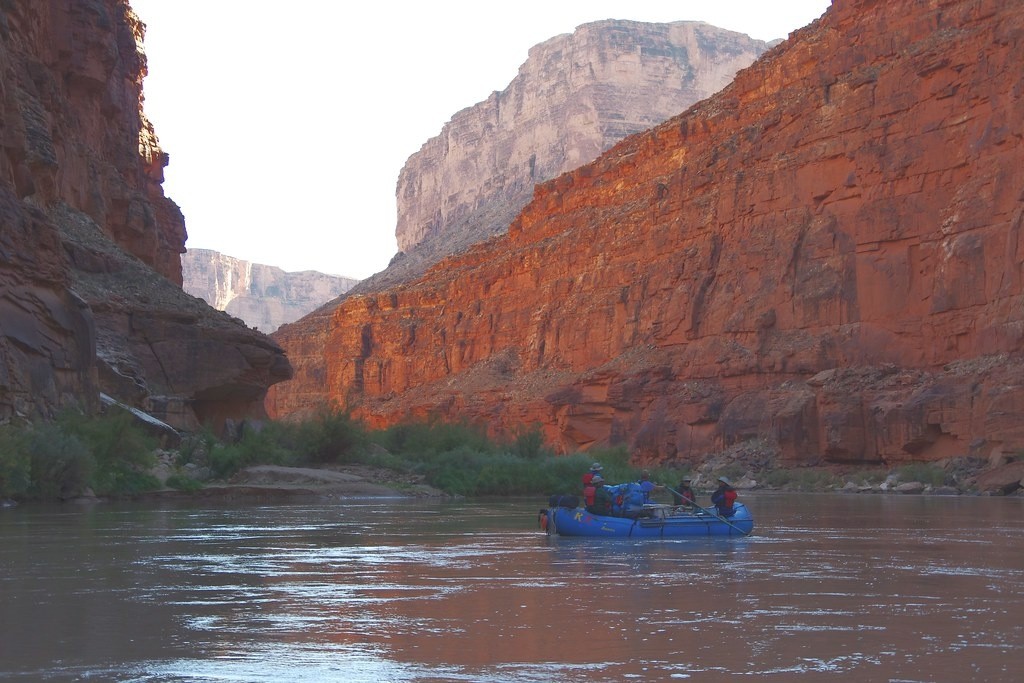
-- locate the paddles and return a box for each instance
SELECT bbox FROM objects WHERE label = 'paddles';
[661,484,750,536]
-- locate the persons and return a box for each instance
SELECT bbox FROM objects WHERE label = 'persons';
[674,475,695,512]
[582,462,666,520]
[711,476,737,518]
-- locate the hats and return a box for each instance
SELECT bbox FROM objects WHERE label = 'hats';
[590,463,603,471]
[590,476,604,485]
[680,476,691,481]
[717,476,730,487]
